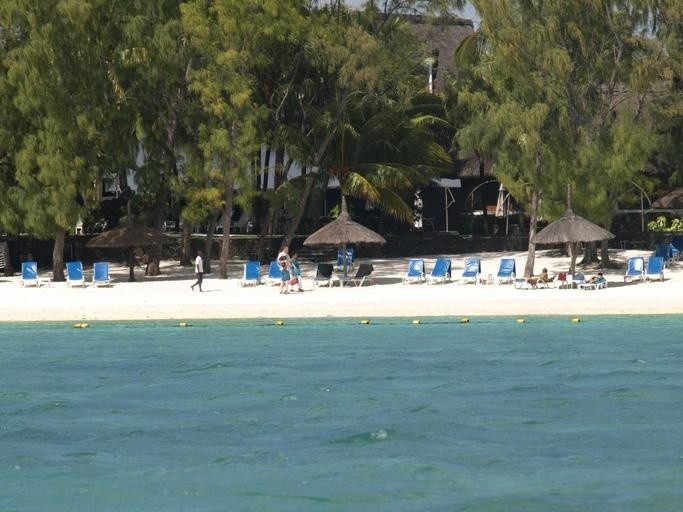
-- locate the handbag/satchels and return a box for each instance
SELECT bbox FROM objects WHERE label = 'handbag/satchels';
[288,277,299,286]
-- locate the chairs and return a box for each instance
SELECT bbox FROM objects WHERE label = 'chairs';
[92,262,110,287]
[402,242,683,290]
[65,261,85,289]
[265,260,283,286]
[19,260,38,288]
[238,260,261,289]
[312,246,375,290]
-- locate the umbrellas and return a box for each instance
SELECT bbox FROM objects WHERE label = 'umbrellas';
[495,182,507,219]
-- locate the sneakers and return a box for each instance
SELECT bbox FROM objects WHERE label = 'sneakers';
[199,290,204,292]
[279,291,283,294]
[297,288,305,292]
[191,286,194,291]
[284,290,290,294]
[290,289,294,292]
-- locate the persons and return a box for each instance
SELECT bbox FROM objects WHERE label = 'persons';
[192,253,208,287]
[277,245,292,294]
[527,269,548,283]
[290,252,304,292]
[585,273,602,284]
